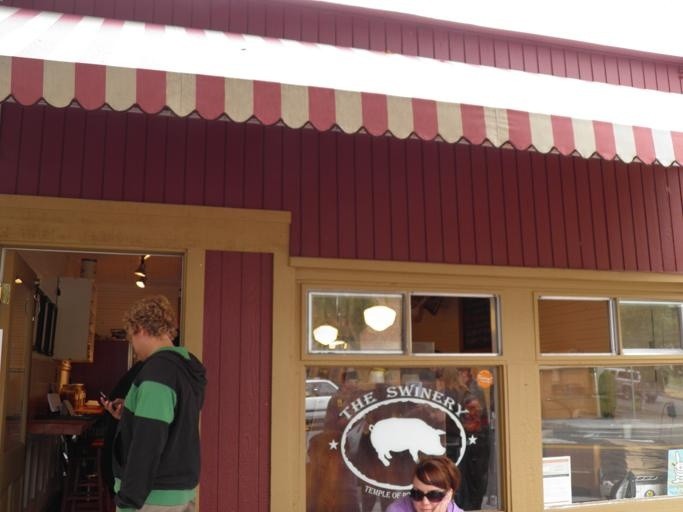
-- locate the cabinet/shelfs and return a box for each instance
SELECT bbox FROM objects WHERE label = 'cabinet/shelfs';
[52,276,94,364]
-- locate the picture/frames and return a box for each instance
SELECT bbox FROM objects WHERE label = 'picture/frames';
[458,297,492,353]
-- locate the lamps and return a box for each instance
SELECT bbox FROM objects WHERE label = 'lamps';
[136,277,145,288]
[134,256,145,277]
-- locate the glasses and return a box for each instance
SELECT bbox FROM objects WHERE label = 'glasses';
[410,489,445,503]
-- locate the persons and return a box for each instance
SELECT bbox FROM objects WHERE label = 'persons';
[99,295,207,511]
[100,337,180,503]
[325,365,492,511]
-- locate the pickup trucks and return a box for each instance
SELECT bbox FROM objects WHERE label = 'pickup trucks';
[600,368,662,403]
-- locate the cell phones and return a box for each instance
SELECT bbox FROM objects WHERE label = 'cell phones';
[99,391,117,411]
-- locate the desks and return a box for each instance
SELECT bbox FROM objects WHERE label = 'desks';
[27,408,103,435]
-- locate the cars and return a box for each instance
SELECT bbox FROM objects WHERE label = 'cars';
[304,378,340,422]
[304,366,492,508]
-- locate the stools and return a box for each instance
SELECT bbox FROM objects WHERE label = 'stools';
[61,439,112,512]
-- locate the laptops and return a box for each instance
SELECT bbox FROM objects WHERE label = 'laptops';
[63,400,86,416]
